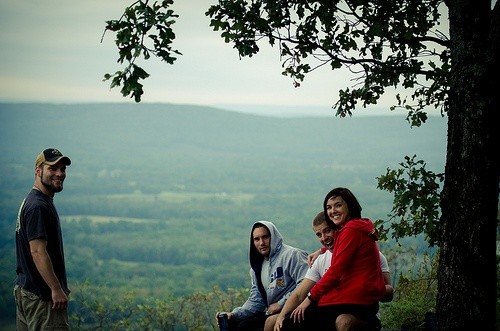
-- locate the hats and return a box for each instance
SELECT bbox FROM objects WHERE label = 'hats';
[35,148,71,169]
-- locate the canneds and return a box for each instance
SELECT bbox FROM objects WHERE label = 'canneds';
[218,313,228,331]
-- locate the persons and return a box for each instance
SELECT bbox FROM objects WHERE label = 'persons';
[261,212,394,331]
[279,186,387,331]
[215,218,313,331]
[13,145,74,331]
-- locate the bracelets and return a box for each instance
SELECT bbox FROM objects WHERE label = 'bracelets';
[308,292,314,305]
